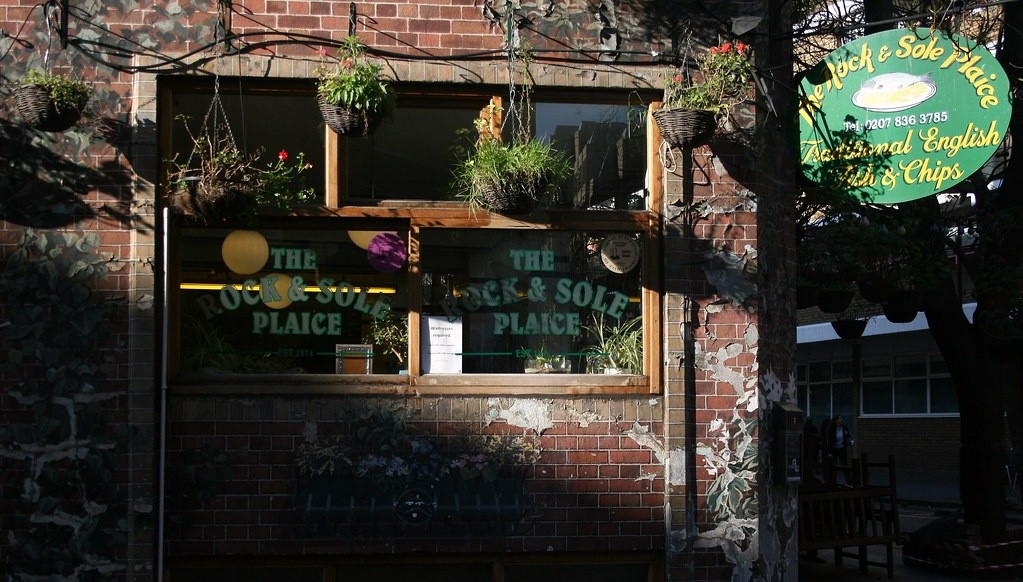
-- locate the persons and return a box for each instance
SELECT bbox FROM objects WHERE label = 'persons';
[821,414,849,481]
[804,416,820,480]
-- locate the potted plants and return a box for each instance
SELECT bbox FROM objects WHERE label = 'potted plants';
[453,129,577,220]
[832,292,871,341]
[14,66,90,134]
[878,262,919,322]
[581,313,645,374]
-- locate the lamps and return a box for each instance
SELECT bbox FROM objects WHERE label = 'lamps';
[221,230,270,276]
[347,231,397,251]
[259,273,295,310]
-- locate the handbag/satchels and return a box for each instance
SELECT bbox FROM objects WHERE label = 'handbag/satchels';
[846,436,854,445]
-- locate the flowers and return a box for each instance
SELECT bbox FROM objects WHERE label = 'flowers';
[317,40,392,114]
[294,425,526,485]
[175,137,312,209]
[666,37,756,105]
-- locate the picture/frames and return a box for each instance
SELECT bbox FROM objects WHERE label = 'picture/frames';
[335,344,373,374]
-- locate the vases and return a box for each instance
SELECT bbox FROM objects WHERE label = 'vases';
[654,106,721,145]
[316,90,385,136]
[312,474,528,522]
[172,152,273,217]
